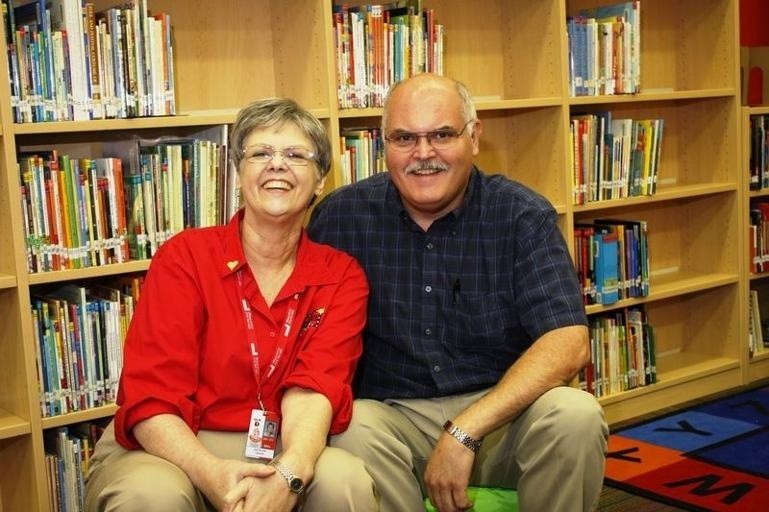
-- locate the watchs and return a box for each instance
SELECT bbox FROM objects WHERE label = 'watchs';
[268,458,305,497]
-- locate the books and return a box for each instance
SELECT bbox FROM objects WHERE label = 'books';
[331,2,446,110]
[340,126,390,184]
[565,1,665,398]
[31,276,141,511]
[748,113,769,357]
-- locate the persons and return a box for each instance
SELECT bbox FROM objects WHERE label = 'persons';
[82,96,381,512]
[305,71,610,512]
[264,421,275,438]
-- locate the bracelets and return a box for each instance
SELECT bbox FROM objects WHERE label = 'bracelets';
[442,419,483,452]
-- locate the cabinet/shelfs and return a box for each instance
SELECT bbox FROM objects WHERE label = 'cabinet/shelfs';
[1,0,769,512]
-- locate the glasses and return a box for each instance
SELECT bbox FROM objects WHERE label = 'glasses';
[384,118,475,153]
[240,143,323,169]
